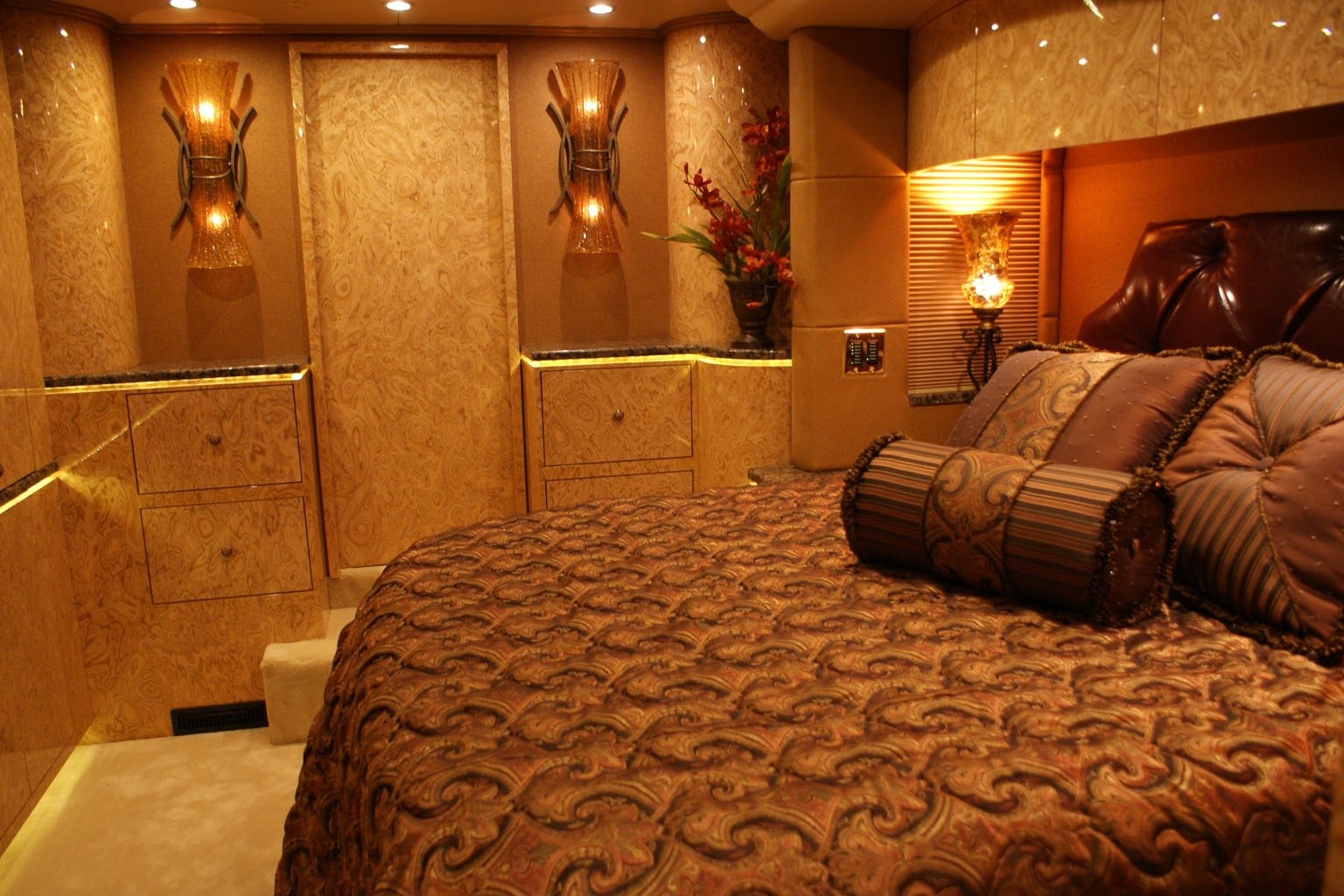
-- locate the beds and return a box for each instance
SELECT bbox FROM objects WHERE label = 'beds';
[271,207,1343,896]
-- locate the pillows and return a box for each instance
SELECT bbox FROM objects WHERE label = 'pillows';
[838,431,1177,632]
[936,336,1245,489]
[1148,341,1344,660]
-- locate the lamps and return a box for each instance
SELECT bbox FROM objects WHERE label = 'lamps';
[159,59,266,271]
[950,210,1022,393]
[549,58,631,255]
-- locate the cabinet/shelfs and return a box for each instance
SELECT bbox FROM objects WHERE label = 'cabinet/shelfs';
[537,371,694,509]
[123,387,315,605]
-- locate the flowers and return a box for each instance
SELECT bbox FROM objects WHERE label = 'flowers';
[637,102,797,311]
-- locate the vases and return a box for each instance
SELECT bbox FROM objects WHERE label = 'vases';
[723,278,779,349]
